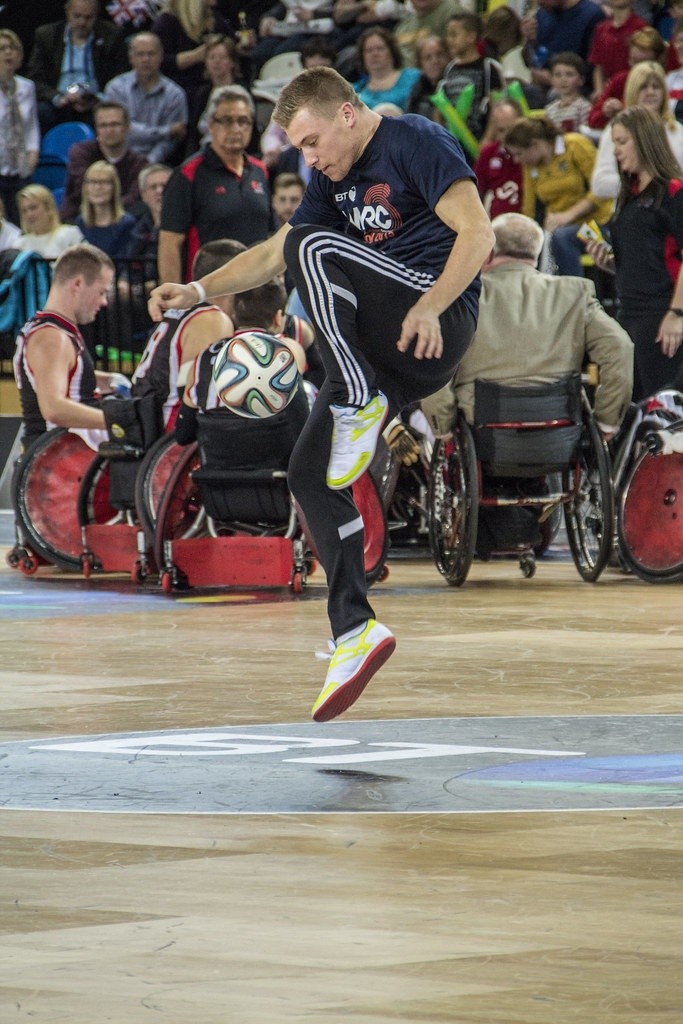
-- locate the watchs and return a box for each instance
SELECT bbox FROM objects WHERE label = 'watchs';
[669,307,683,318]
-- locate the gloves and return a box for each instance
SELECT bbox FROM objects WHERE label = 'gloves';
[383,415,424,467]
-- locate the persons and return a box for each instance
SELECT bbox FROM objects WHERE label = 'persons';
[148,66,496,724]
[0,0,683,453]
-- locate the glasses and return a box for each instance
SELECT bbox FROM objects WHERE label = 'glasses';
[212,113,256,126]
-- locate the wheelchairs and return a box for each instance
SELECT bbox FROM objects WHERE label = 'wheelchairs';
[425,377,683,587]
[7,388,564,597]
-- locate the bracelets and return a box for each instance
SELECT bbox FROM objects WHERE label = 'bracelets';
[188,281,206,306]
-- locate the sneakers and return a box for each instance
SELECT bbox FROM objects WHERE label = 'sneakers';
[308,617,396,722]
[324,387,390,491]
